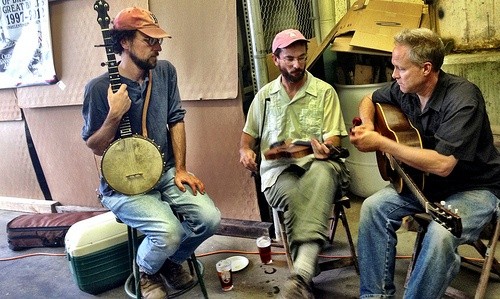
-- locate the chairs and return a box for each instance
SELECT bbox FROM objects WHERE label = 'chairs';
[276,195,360,276]
[403,213,500,299]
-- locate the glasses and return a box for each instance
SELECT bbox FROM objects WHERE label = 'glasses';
[135,35,163,46]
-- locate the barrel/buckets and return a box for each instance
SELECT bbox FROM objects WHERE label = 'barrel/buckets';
[334,80,396,198]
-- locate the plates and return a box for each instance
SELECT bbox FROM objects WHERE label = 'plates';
[226,256,249,271]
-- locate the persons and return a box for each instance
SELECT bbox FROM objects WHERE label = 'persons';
[240,29,351,299]
[349,27,500,299]
[82,5,222,299]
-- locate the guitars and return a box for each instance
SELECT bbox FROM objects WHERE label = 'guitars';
[373,102,463,240]
[93,0,166,195]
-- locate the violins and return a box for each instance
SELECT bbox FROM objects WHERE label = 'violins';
[263,138,350,160]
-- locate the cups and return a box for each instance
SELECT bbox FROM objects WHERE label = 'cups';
[256,236,272,264]
[216,260,234,291]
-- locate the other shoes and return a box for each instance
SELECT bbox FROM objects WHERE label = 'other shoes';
[276,273,316,299]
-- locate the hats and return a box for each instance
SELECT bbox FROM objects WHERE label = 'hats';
[110,6,172,39]
[272,28,311,53]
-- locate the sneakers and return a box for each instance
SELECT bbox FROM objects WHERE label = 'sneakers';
[139,272,169,299]
[160,258,196,290]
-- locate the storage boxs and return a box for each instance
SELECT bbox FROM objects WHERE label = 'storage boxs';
[63,210,148,296]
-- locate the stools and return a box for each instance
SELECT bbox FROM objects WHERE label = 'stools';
[114,216,209,299]
